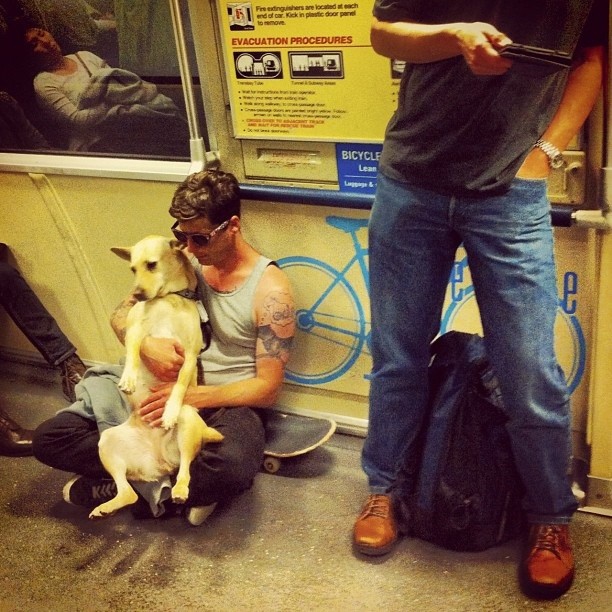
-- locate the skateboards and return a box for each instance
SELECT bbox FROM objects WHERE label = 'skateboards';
[258,409,336,473]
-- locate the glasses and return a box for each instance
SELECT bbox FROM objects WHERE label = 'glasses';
[172,220,230,245]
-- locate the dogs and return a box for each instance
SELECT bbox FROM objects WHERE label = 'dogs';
[86,234,226,522]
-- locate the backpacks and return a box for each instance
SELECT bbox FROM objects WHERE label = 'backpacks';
[401,330,525,553]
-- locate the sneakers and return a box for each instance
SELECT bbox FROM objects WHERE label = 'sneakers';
[184,501,218,526]
[63,474,117,509]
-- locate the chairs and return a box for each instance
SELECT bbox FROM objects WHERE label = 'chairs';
[0,89,53,151]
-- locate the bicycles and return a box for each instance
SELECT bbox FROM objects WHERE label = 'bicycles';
[273,217,586,395]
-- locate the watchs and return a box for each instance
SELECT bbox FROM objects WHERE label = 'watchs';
[535,138,566,170]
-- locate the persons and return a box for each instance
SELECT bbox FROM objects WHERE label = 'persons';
[355,1,611,599]
[17,21,190,153]
[29,171,294,526]
[0,242,90,455]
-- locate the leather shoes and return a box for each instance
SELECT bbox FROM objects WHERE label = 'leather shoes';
[61,352,88,403]
[520,522,574,601]
[354,492,399,556]
[1,409,36,457]
